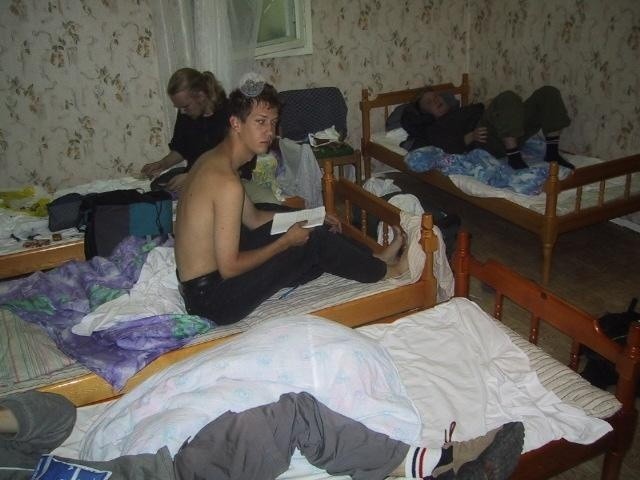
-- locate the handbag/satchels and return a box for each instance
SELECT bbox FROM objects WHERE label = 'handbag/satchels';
[576,312,640,389]
[46,188,173,260]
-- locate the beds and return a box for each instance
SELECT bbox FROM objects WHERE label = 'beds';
[43,231,640,480]
[1,159,442,409]
[359,73,640,284]
[0,128,324,279]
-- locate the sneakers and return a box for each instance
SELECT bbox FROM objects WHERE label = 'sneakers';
[422,422,525,480]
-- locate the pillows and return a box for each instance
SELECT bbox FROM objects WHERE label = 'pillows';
[386,127,409,144]
[386,104,408,129]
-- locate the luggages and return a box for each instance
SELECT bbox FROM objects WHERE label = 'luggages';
[350,183,459,259]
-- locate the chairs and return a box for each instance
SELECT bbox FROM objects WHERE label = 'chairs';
[276,87,361,182]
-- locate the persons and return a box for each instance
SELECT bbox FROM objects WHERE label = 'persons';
[140,67,258,201]
[175,81,413,327]
[419,86,574,173]
[0,384,523,480]
